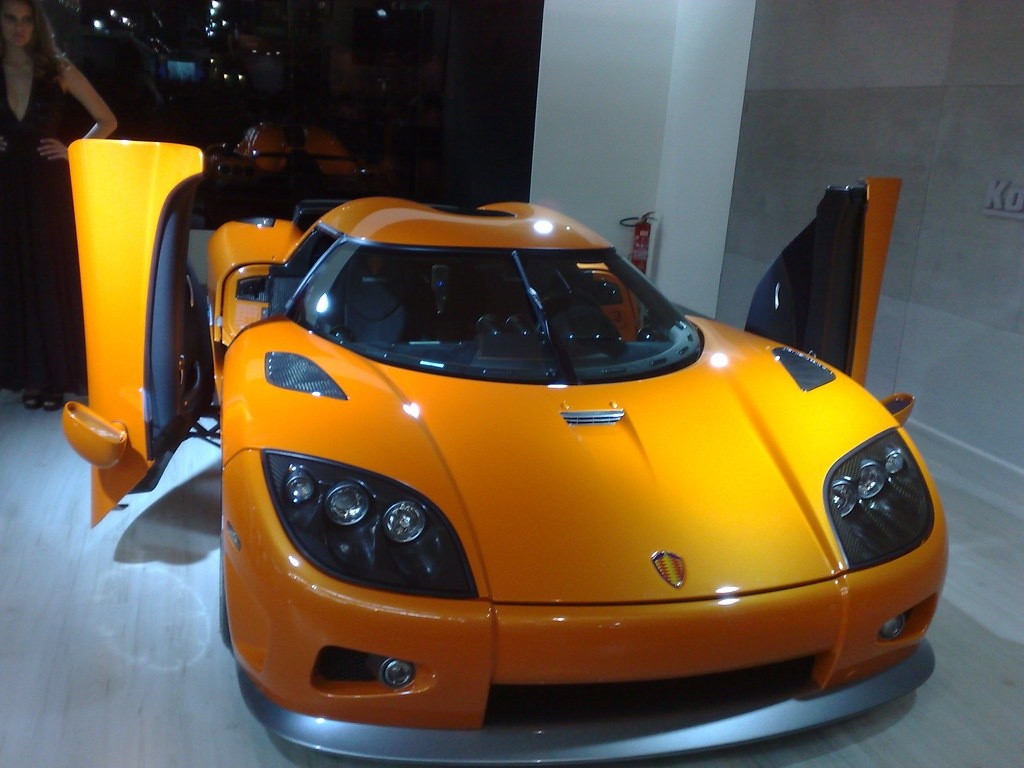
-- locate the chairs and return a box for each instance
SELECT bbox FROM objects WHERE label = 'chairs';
[347,276,407,339]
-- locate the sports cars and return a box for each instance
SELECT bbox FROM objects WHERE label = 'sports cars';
[62,139,950,766]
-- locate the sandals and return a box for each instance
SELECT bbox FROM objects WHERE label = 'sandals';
[22,382,64,411]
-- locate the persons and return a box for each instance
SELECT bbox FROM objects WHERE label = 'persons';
[0,0,117,412]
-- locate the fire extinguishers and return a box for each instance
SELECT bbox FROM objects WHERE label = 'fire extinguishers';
[619,209,659,275]
